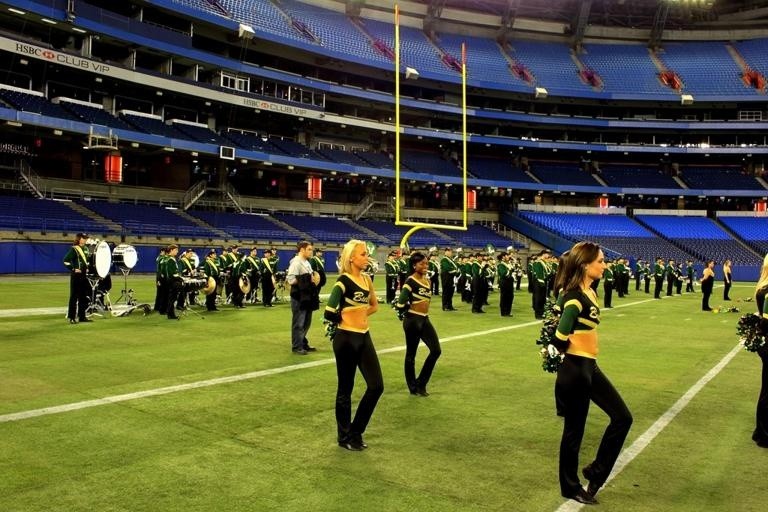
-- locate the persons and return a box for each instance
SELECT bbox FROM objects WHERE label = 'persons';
[324,240,384,450]
[686,260,695,292]
[429,248,560,319]
[154,245,179,318]
[611,257,631,298]
[309,248,326,303]
[643,261,651,293]
[737,254,768,448]
[542,241,633,504]
[225,244,244,304]
[217,249,229,298]
[700,260,715,310]
[270,248,281,301]
[660,258,665,291]
[246,247,261,303]
[676,263,683,294]
[395,253,441,396]
[204,249,220,311]
[231,251,250,309]
[552,251,570,415]
[604,261,614,308]
[260,249,276,307]
[287,241,320,354]
[635,258,643,291]
[722,260,732,300]
[176,249,196,310]
[64,233,94,324]
[384,249,408,304]
[665,258,677,296]
[654,257,664,299]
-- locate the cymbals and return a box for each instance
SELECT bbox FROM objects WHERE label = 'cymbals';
[239,276,250,294]
[271,274,277,289]
[200,276,216,296]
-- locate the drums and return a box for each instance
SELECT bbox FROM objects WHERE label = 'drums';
[112,243,138,271]
[86,241,112,281]
[179,251,200,268]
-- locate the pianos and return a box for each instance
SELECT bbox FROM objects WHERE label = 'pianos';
[181,278,207,292]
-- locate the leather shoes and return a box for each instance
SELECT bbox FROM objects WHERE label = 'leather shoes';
[751,436,757,441]
[575,487,597,504]
[239,305,245,308]
[472,310,479,313]
[79,318,92,322]
[449,307,458,311]
[583,465,601,497]
[293,347,306,354]
[168,316,178,319]
[418,379,429,397]
[351,430,366,448]
[255,298,261,302]
[443,307,451,311]
[69,318,75,324]
[756,441,768,448]
[176,306,183,310]
[504,314,514,317]
[209,308,219,312]
[478,309,485,313]
[339,437,360,450]
[304,344,315,351]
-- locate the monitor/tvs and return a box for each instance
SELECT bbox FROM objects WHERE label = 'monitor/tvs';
[219,146,235,160]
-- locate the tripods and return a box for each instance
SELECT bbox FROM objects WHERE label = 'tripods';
[174,293,205,320]
[113,269,133,305]
[271,281,288,304]
[83,278,105,317]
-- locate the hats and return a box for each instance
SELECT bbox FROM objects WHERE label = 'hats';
[668,258,674,261]
[541,250,551,255]
[388,253,396,256]
[262,249,271,253]
[445,247,453,251]
[656,257,662,260]
[645,262,650,264]
[208,249,216,254]
[231,244,237,249]
[250,247,256,251]
[316,248,323,252]
[77,232,88,238]
[637,258,642,260]
